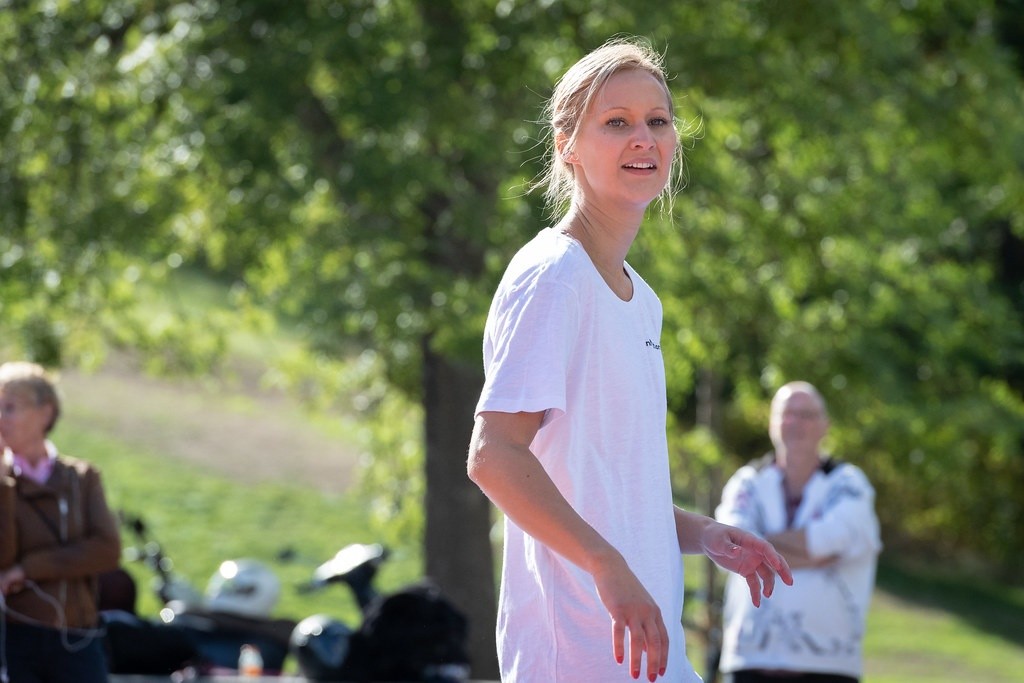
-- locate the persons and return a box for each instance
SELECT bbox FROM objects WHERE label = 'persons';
[468,39,793,683]
[1,362,124,680]
[717,383,881,683]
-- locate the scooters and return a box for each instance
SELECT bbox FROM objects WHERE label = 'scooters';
[93,509,471,683]
